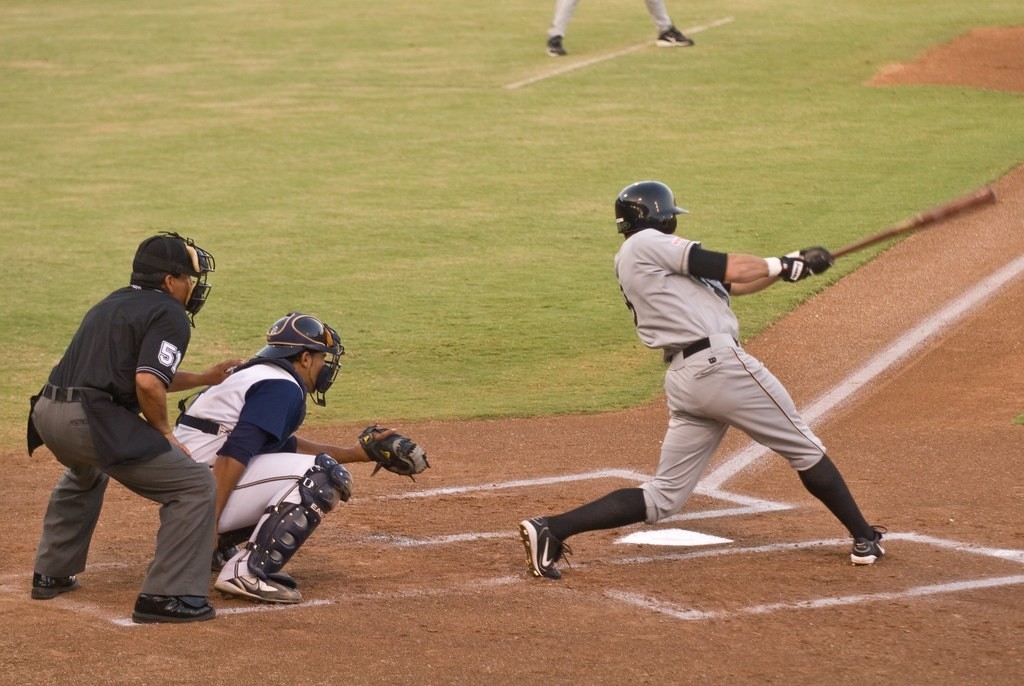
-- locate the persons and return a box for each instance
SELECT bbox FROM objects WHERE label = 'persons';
[520,181,888,578]
[27,228,245,624]
[173,312,431,604]
[545,0,694,57]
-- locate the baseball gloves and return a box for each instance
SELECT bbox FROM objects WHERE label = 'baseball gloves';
[357,424,432,484]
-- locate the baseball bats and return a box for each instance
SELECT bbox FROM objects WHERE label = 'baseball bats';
[830,185,1001,258]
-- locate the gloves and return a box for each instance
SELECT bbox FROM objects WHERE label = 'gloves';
[764,247,831,283]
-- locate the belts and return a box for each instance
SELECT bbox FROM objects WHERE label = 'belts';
[41,384,85,402]
[664,335,739,367]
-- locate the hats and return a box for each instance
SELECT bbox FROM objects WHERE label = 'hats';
[133,235,191,274]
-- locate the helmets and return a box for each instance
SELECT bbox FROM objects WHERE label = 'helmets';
[255,313,328,358]
[615,181,689,234]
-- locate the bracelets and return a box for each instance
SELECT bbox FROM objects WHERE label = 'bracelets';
[765,257,782,276]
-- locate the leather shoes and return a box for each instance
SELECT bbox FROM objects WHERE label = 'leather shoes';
[32,571,79,598]
[131,595,217,625]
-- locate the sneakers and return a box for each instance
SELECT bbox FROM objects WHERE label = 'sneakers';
[517,514,572,579]
[546,34,568,56]
[655,25,696,48]
[215,573,302,604]
[211,549,228,571]
[849,525,887,566]
